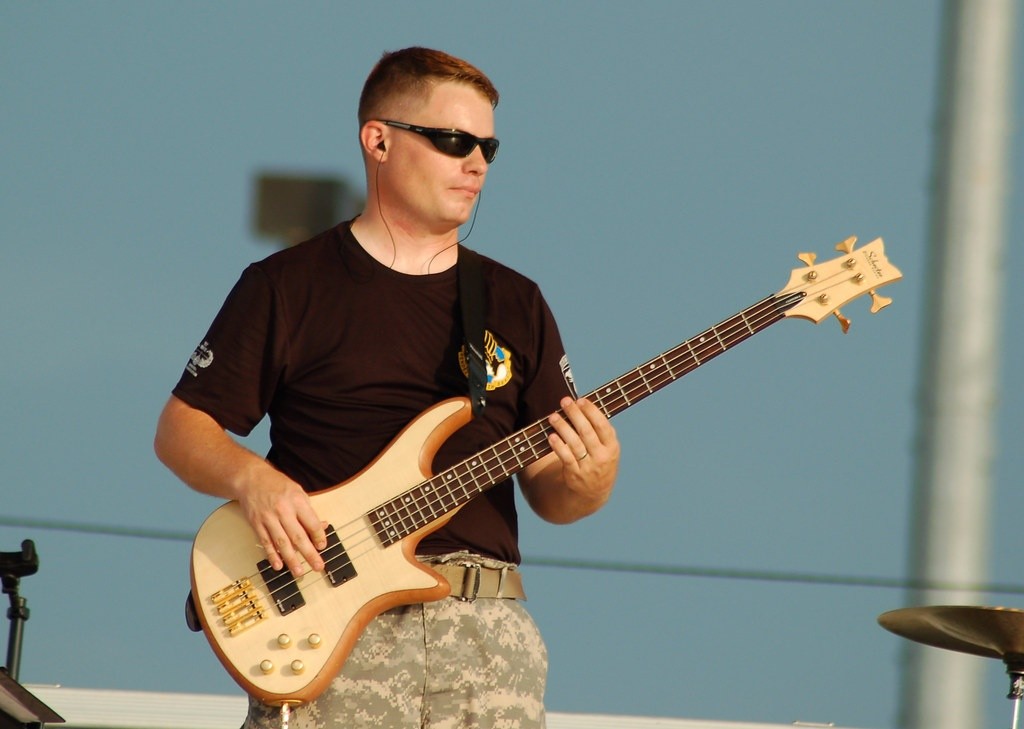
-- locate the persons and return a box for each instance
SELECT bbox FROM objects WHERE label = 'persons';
[153,47,619,729]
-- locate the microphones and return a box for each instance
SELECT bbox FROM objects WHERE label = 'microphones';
[454,255,489,412]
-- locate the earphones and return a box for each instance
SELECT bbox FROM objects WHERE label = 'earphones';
[377,141,386,150]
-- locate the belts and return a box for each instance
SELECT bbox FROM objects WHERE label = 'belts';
[422,562,528,603]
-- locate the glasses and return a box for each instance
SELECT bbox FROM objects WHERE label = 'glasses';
[380,120,499,164]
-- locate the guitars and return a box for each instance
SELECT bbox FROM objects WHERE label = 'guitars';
[186,231,907,714]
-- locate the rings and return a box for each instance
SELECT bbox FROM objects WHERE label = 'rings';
[577,453,588,461]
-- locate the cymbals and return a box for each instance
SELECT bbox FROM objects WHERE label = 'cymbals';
[873,603,1023,659]
